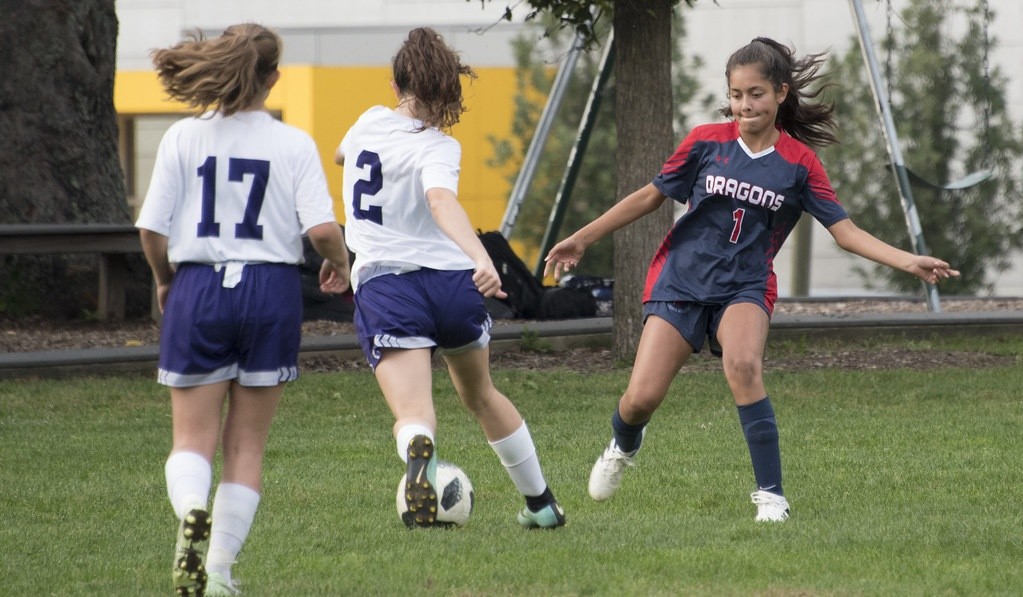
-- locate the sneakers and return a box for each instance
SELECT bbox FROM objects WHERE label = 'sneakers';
[588,426,647,501]
[172,508,212,597]
[750,490,789,524]
[517,496,567,530]
[405,434,439,525]
[201,573,242,597]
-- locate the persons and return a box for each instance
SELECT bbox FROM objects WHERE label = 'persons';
[336,28,564,528]
[134,24,350,597]
[544,38,962,521]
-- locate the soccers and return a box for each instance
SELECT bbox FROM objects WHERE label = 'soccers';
[396,458,475,528]
